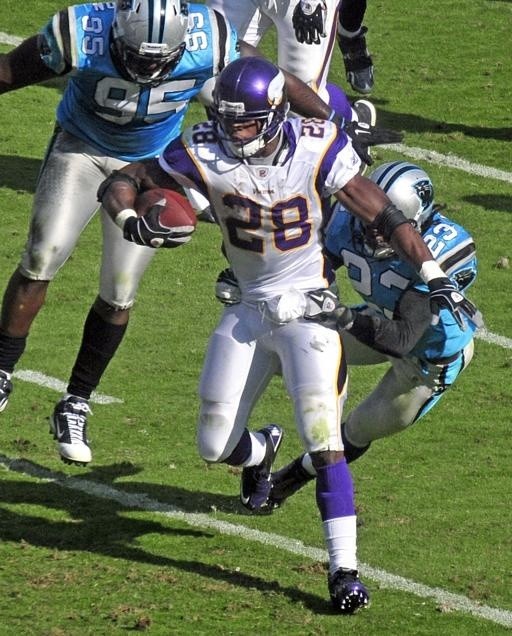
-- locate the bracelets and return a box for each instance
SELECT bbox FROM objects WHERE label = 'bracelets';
[115,208,139,232]
[414,259,450,284]
[327,110,345,130]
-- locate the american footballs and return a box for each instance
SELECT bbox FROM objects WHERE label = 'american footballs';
[135,189,197,229]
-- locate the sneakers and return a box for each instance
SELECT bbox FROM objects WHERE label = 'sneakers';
[0,368,13,412]
[49,394,93,468]
[239,423,284,511]
[263,453,315,509]
[183,186,214,222]
[328,568,370,615]
[337,24,374,97]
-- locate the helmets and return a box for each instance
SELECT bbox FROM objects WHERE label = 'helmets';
[350,159,435,260]
[212,56,287,161]
[109,0,189,89]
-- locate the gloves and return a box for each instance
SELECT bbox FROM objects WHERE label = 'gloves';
[304,288,355,332]
[291,0,326,45]
[123,198,195,249]
[214,267,242,306]
[339,118,404,166]
[425,276,484,333]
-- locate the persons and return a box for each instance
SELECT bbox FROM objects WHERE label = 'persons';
[1,0,406,465]
[219,0,343,94]
[334,0,378,95]
[212,158,479,519]
[93,55,490,618]
